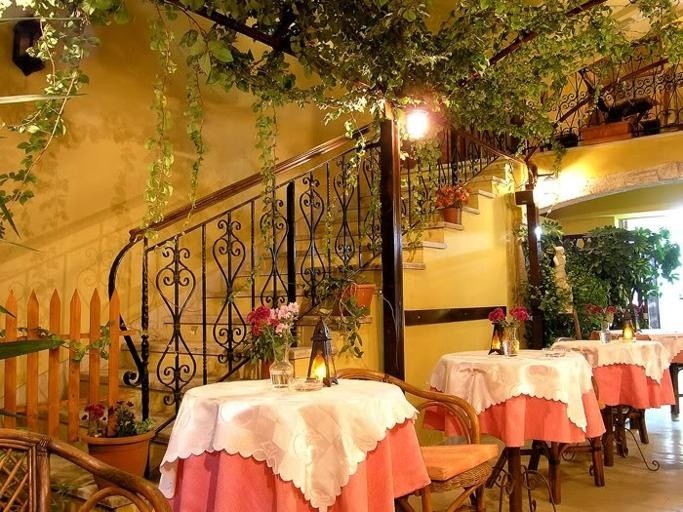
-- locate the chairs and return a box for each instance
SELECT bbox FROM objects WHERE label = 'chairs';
[1,428,172,512]
[336,368,500,512]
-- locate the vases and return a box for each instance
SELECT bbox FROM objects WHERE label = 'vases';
[507,327,519,356]
[81,428,156,495]
[269,337,294,388]
[599,321,611,343]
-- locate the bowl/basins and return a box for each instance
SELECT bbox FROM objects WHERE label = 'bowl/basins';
[617,336,638,346]
[284,375,325,393]
[541,345,567,361]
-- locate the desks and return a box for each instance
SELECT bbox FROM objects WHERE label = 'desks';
[609,330,683,421]
[551,338,677,466]
[157,378,431,512]
[422,348,605,511]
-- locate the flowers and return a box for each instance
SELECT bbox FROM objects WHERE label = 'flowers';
[246,303,299,343]
[79,400,153,438]
[586,304,615,322]
[488,305,529,326]
